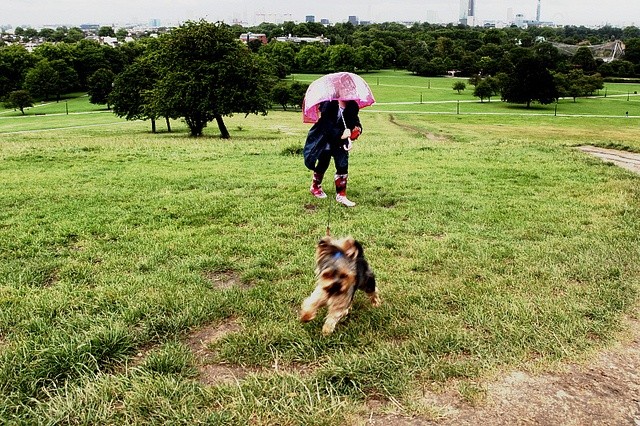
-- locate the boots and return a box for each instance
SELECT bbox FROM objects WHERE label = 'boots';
[309,169,328,199]
[335,173,355,206]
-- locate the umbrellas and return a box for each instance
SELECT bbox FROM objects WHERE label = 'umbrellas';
[302,72,375,151]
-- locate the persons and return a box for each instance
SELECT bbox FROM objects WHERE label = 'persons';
[303,100,363,206]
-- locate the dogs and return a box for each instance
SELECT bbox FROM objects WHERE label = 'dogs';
[301,234,383,337]
